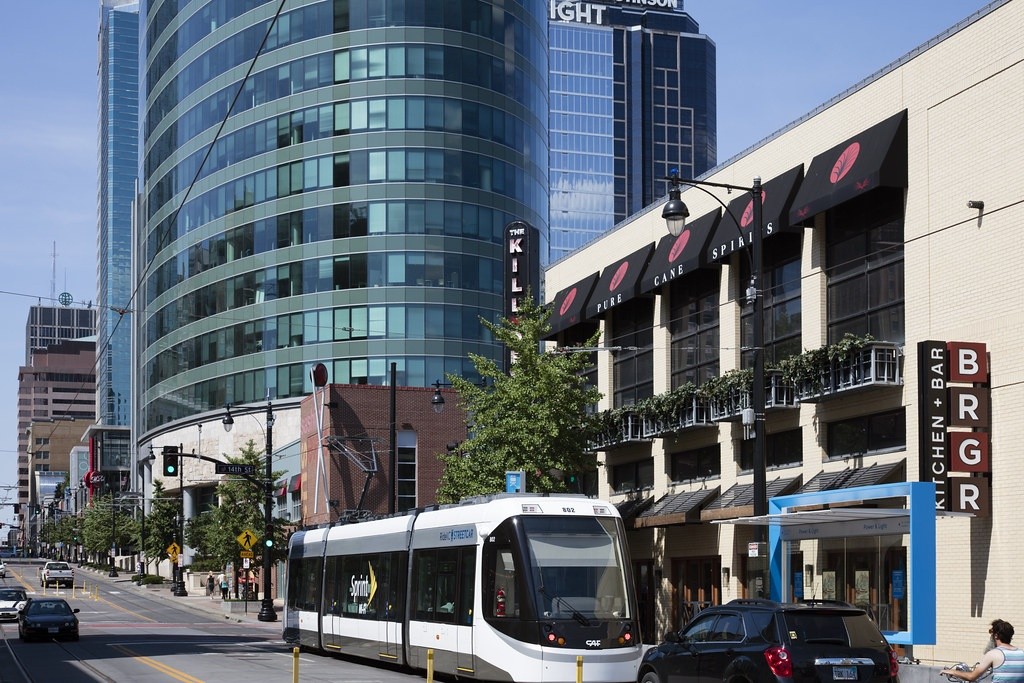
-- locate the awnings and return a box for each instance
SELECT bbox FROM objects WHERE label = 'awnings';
[585,241,655,320]
[788,108,908,225]
[640,206,722,295]
[540,271,600,341]
[705,163,804,264]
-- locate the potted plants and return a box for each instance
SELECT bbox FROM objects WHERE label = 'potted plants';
[585,332,891,450]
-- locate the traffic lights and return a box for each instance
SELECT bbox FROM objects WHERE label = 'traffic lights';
[163,447,179,476]
[35,504,40,514]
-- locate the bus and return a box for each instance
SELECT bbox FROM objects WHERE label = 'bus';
[281,492,642,683]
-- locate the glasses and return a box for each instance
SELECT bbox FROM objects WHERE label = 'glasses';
[989,629,995,634]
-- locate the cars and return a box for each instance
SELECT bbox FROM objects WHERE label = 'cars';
[0,586,32,623]
[18,598,80,643]
[0,559,7,578]
[637,597,901,683]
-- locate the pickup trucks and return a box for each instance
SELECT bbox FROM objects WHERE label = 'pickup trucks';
[39,562,74,589]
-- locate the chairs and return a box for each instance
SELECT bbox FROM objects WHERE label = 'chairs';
[54,605,62,612]
[416,278,454,288]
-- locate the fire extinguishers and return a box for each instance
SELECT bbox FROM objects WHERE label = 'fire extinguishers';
[496,584,506,617]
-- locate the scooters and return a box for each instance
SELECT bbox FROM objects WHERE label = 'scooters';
[944,662,993,683]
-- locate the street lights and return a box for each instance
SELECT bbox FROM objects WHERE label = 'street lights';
[430,375,491,416]
[105,482,118,577]
[655,168,769,543]
[137,454,156,586]
[147,443,188,596]
[222,401,278,622]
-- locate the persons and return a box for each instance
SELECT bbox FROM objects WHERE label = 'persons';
[939,619,1024,683]
[205,571,224,600]
[220,575,228,600]
[136,561,141,575]
